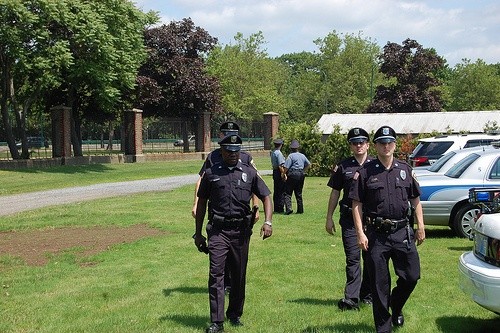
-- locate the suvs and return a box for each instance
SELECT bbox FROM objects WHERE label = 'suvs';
[408,134,500,169]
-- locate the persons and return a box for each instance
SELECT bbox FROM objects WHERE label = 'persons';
[270,139,287,213]
[326,128,376,310]
[349,126,425,333]
[195,134,273,333]
[281,140,310,215]
[192,122,259,295]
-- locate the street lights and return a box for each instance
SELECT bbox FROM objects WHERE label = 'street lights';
[371,61,387,105]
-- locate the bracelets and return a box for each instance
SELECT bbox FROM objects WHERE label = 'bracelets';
[264,221,272,227]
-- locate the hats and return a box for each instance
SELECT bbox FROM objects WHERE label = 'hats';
[290,140,299,148]
[219,121,239,136]
[218,135,242,151]
[273,139,282,145]
[347,127,369,142]
[373,126,396,143]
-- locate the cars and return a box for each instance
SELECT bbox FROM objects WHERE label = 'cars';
[173,135,195,147]
[16,137,44,149]
[413,139,500,238]
[458,212,500,316]
[412,145,492,179]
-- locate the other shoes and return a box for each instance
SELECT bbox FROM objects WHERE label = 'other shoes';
[286,209,293,215]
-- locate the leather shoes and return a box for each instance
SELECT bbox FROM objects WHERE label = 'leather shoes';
[338,298,360,311]
[206,321,224,333]
[392,308,404,326]
[363,299,371,304]
[230,316,242,326]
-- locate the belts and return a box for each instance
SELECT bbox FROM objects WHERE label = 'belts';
[338,202,353,218]
[364,216,408,232]
[224,218,245,226]
